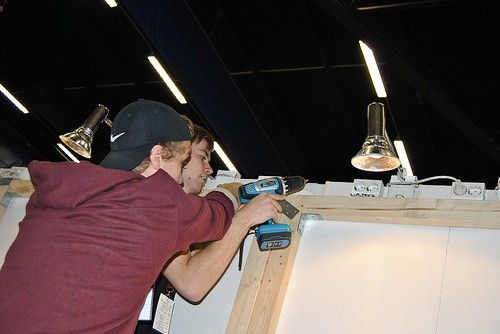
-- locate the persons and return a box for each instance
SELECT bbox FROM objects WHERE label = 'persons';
[134,126,287,334]
[0,99,248,334]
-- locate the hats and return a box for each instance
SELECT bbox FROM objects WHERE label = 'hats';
[99,99,193,170]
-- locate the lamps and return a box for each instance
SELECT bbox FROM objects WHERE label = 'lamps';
[350,102,401,172]
[59,104,113,158]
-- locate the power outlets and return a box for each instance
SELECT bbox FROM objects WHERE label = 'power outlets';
[451,182,486,200]
[9,167,28,180]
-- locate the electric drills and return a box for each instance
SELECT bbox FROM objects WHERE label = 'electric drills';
[238,174,310,252]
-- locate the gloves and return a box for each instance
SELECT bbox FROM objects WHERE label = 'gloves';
[212,182,243,213]
